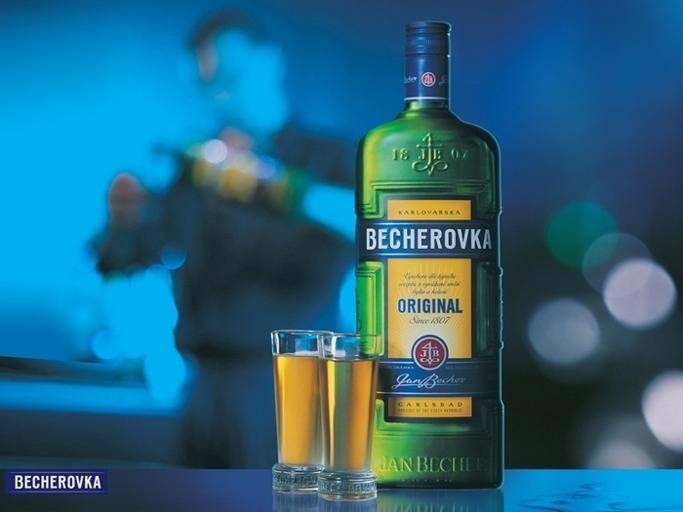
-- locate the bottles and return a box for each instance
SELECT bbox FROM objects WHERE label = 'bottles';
[355,21,507,489]
[151,138,309,216]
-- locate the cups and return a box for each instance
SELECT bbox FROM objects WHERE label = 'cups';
[263,326,379,503]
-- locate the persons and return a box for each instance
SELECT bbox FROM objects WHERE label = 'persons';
[90,17,358,467]
